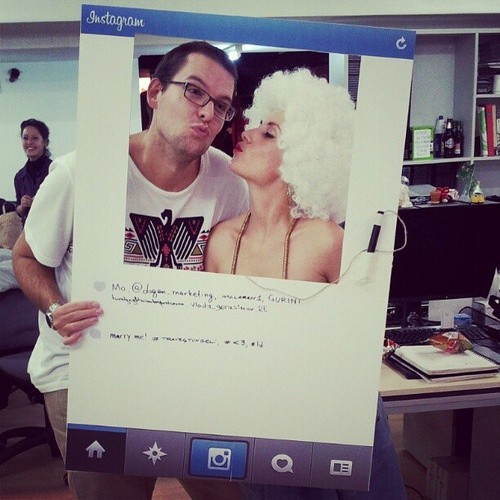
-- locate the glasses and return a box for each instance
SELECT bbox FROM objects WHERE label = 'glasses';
[160,80,235,122]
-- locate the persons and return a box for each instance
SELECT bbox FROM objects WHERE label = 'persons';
[204,67,405,500]
[14,119,53,219]
[14,42,249,500]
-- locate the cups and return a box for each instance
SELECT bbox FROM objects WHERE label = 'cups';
[430,191,441,204]
[492,74,500,93]
[454,313,471,327]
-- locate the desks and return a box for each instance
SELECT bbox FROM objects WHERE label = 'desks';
[378,325,500,500]
[388,199,500,305]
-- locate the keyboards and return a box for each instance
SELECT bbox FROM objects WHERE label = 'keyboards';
[385,326,492,347]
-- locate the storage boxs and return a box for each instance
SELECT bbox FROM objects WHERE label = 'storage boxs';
[423,455,468,500]
[409,125,435,161]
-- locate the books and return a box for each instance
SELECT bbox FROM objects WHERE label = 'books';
[478,103,497,157]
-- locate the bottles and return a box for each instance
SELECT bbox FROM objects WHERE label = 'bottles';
[433,114,446,157]
[442,118,455,157]
[454,120,462,157]
[453,120,457,132]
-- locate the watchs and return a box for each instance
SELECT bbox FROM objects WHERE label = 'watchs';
[46,302,60,328]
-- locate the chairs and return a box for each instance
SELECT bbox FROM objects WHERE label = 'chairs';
[0,289,59,466]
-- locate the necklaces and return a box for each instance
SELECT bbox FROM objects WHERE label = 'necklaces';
[229,211,297,280]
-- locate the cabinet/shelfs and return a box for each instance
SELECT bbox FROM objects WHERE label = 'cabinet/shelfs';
[402,27,500,167]
